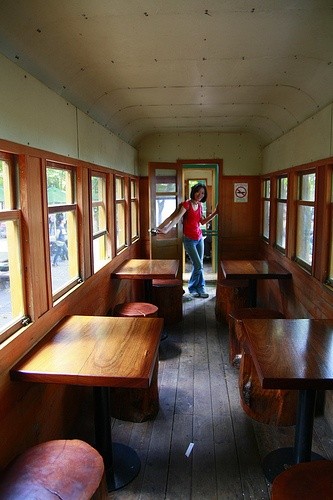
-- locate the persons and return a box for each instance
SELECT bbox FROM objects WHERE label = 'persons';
[156,183,218,298]
[49,215,69,266]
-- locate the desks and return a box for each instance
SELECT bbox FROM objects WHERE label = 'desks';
[112,259,182,340]
[241,318,333,489]
[221,260,292,307]
[8,314,164,492]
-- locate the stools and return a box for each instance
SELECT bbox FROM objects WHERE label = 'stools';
[269,461,333,500]
[111,355,159,421]
[214,277,301,424]
[113,302,158,318]
[0,440,108,500]
[152,279,183,323]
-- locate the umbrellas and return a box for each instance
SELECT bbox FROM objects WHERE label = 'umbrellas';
[46,186,66,235]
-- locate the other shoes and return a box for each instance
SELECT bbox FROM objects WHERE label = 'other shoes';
[199,293,209,298]
[190,292,199,297]
